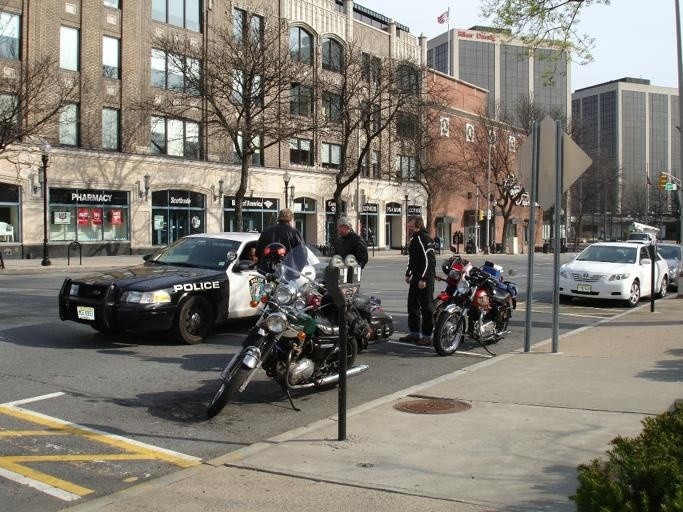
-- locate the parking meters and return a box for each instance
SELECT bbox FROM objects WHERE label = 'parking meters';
[323,252,361,438]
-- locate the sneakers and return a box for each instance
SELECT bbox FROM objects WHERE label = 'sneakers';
[398,334,420,343]
[415,337,431,346]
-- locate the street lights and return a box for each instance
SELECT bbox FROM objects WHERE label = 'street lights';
[37,138,53,268]
[402,185,411,255]
[283,167,296,224]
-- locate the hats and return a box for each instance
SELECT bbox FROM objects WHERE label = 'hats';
[274,208,292,223]
[337,217,352,229]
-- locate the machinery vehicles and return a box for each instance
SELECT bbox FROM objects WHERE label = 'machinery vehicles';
[624,221,661,246]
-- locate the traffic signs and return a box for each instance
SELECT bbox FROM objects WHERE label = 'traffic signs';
[665,183,677,190]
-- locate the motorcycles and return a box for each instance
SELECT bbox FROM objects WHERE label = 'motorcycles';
[202,240,398,420]
[431,247,518,354]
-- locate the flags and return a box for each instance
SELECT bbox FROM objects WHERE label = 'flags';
[438,11,448,25]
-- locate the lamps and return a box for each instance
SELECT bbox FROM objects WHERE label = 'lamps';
[33,164,44,194]
[212,178,225,204]
[140,172,150,202]
[288,183,295,201]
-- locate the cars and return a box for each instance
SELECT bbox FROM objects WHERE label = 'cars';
[56,233,360,342]
[557,240,683,308]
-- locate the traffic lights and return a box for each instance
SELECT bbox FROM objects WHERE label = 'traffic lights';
[658,174,668,191]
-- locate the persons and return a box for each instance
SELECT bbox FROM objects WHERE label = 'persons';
[254,208,307,286]
[397,213,436,347]
[245,246,258,268]
[331,215,369,273]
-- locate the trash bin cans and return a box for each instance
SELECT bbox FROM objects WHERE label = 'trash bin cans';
[543,242,550,253]
